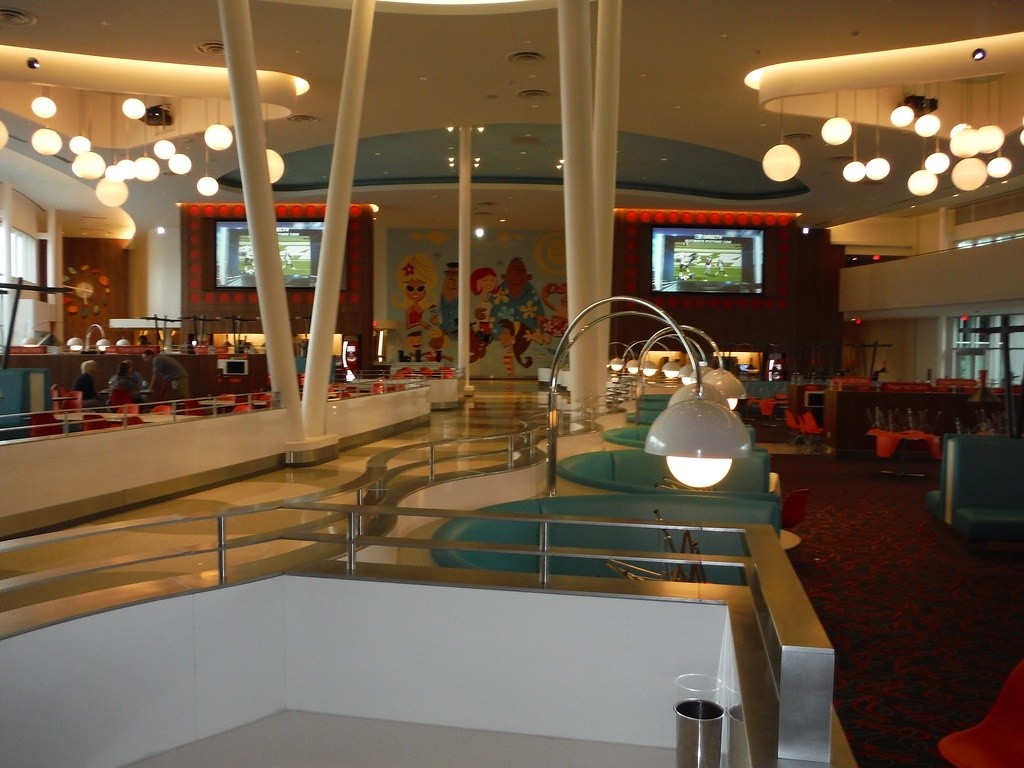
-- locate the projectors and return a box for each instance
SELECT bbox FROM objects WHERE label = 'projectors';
[139,105,171,126]
[897,95,937,117]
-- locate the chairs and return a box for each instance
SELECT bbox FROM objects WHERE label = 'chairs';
[781,488,810,530]
[327,383,405,399]
[939,660,1024,768]
[29,384,275,437]
[745,394,824,450]
[394,366,454,380]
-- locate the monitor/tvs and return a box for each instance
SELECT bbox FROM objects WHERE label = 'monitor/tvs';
[223,360,248,375]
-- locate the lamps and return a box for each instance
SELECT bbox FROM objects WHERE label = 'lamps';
[66,324,132,352]
[31,85,233,208]
[545,294,748,495]
[762,98,801,183]
[821,77,1014,197]
[267,106,285,183]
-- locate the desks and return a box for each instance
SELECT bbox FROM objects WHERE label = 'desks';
[865,404,1011,479]
[54,412,200,424]
[348,378,421,384]
[328,391,371,397]
[200,399,268,406]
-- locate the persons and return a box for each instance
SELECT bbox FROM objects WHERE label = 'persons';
[72,350,189,415]
[138,335,152,346]
[676,252,728,281]
[282,249,296,270]
[244,244,255,275]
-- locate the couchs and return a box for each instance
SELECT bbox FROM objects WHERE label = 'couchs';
[396,490,777,590]
[554,447,773,501]
[605,391,758,449]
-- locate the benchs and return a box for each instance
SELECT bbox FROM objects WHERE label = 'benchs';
[0,515,859,768]
[924,433,1024,555]
